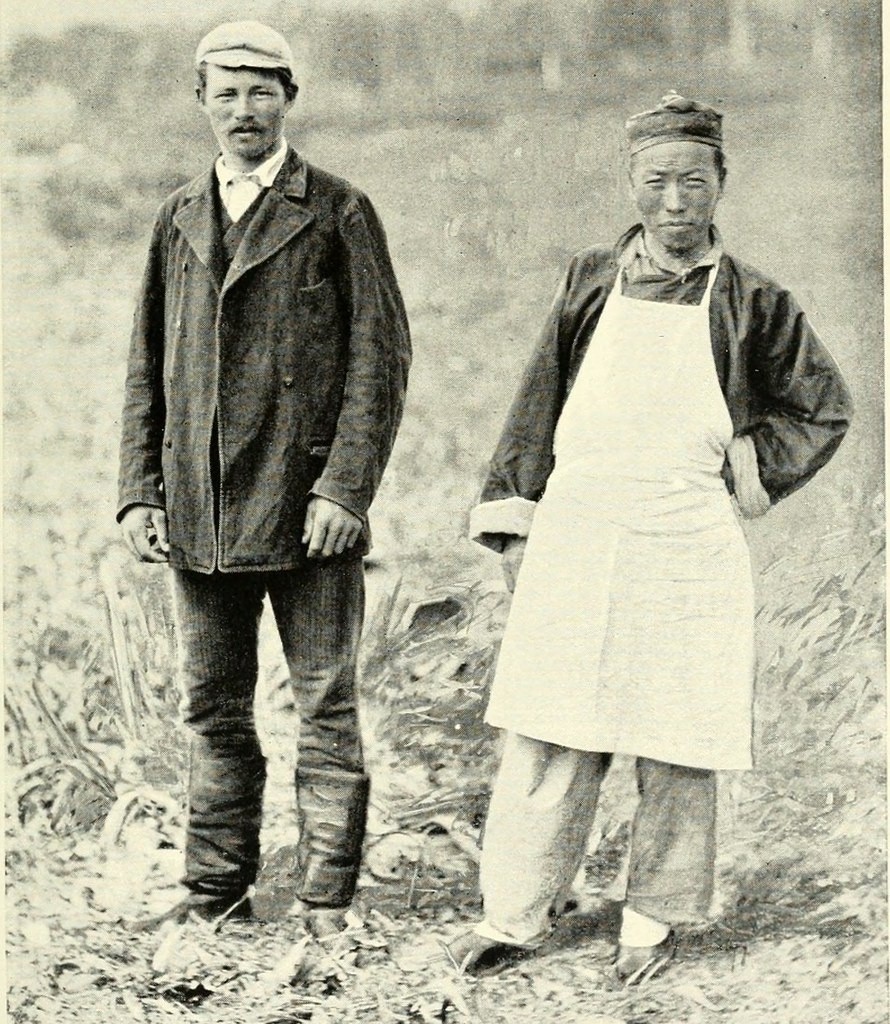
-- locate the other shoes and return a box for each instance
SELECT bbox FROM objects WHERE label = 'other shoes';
[446,920,553,978]
[130,892,253,935]
[613,907,676,985]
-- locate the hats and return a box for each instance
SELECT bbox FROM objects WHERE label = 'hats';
[623,89,723,156]
[195,21,294,78]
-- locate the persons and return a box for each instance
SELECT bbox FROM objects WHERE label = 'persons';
[459,96,855,979]
[116,22,414,986]
